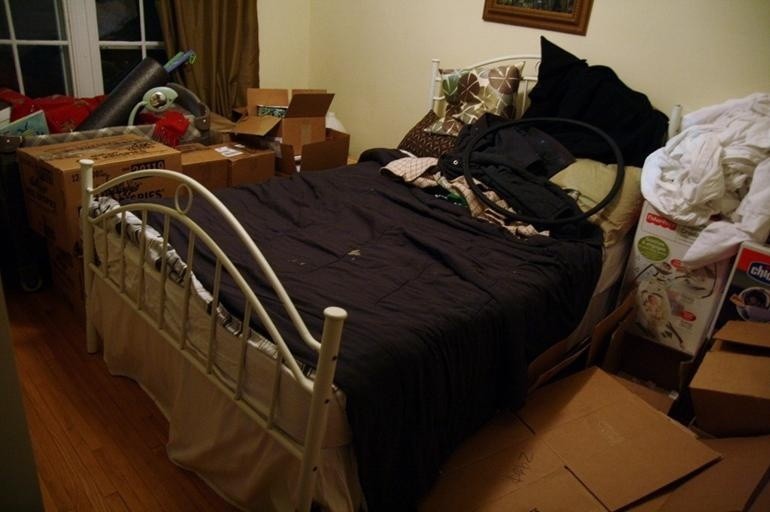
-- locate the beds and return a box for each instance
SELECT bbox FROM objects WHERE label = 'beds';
[78,54,680,512]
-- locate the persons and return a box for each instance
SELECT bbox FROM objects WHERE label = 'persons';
[644,295,661,321]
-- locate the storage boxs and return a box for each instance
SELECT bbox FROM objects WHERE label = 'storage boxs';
[617,200,770,438]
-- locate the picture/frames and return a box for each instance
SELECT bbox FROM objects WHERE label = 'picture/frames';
[481,0,593,36]
